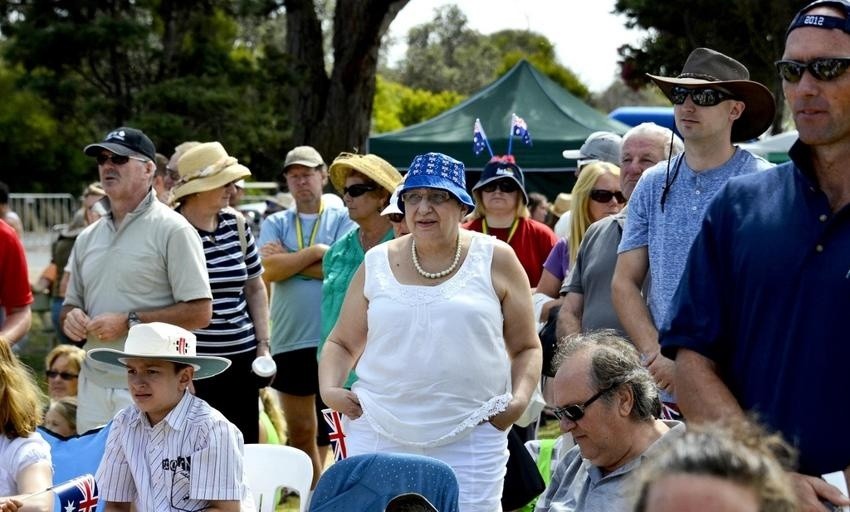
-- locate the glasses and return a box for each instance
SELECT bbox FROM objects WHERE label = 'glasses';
[552,383,615,422]
[591,188,628,204]
[669,85,735,107]
[96,152,148,166]
[341,183,373,197]
[46,369,78,381]
[400,189,452,205]
[773,56,850,84]
[481,179,519,192]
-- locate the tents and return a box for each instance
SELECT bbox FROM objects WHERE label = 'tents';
[365,61,632,177]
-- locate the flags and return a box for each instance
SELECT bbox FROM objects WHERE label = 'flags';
[511,114,532,147]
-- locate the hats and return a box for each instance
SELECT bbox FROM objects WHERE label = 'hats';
[380,173,405,217]
[644,48,776,143]
[87,322,232,380]
[473,161,528,206]
[548,193,571,218]
[397,152,475,218]
[84,128,156,164]
[563,131,623,167]
[329,147,403,194]
[780,2,850,35]
[283,145,324,170]
[166,141,252,206]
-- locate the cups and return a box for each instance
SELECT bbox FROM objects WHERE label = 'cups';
[247,353,278,390]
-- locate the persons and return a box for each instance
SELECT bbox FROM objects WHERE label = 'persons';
[611,45,775,417]
[656,2,850,511]
[0,123,797,510]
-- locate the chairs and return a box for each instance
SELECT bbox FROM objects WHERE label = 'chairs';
[241,443,315,511]
[308,453,459,511]
